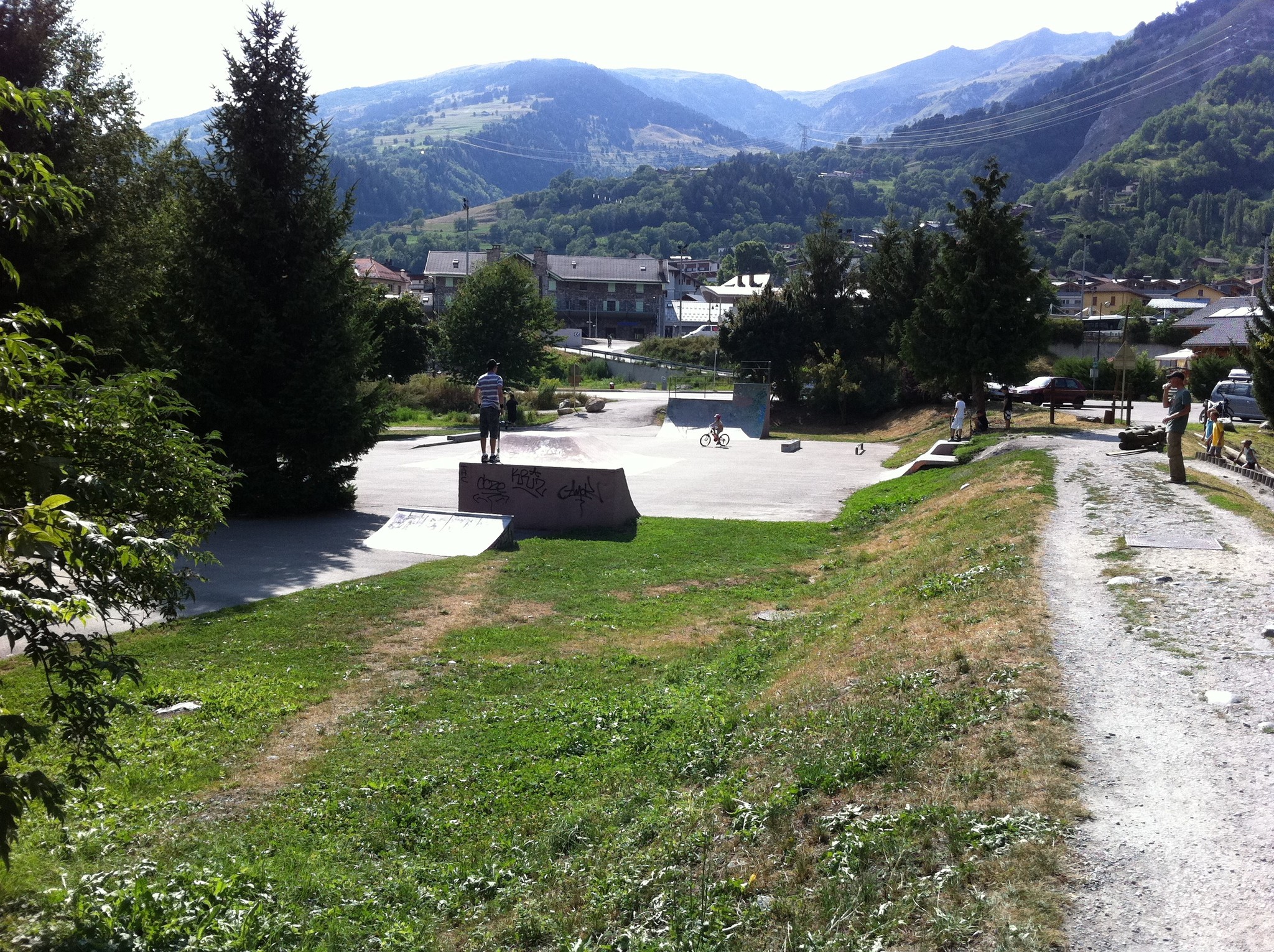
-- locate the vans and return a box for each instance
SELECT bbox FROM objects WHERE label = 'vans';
[681,324,721,339]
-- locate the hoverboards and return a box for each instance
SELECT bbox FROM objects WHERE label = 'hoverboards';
[943,411,972,441]
[486,421,512,463]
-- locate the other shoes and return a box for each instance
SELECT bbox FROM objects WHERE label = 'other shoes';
[948,438,954,441]
[1162,477,1186,485]
[511,428,514,430]
[505,425,508,430]
[717,442,721,445]
[955,438,960,441]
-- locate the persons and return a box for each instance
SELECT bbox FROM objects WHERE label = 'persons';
[1000,386,1012,431]
[474,359,504,463]
[948,393,966,442]
[607,334,612,345]
[1233,438,1261,470]
[505,394,518,430]
[1162,371,1191,485]
[1205,411,1225,458]
[710,414,723,445]
[1201,409,1218,454]
[969,410,988,432]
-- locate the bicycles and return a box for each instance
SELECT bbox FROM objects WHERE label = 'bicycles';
[700,425,730,447]
[607,341,612,348]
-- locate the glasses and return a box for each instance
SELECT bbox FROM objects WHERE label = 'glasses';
[495,366,498,368]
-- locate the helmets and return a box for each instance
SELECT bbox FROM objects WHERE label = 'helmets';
[714,414,721,418]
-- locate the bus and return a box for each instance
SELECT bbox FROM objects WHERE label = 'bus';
[1075,315,1157,339]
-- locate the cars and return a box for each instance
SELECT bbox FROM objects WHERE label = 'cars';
[1007,376,1087,409]
[984,382,1005,402]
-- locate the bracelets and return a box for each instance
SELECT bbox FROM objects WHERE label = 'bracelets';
[478,404,481,408]
[500,404,503,409]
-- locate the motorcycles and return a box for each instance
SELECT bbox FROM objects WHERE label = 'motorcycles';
[1199,389,1234,424]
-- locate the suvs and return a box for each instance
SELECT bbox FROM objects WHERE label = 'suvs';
[1210,368,1270,422]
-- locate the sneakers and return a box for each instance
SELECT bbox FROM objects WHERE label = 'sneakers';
[481,455,490,462]
[490,456,500,462]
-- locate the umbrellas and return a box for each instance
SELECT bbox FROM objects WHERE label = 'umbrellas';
[1155,348,1195,370]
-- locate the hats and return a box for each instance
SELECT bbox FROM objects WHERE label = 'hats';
[1241,440,1252,445]
[1166,370,1185,380]
[487,359,500,367]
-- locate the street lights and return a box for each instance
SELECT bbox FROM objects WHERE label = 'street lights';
[708,296,719,326]
[1094,301,1111,397]
[462,197,470,289]
[837,228,854,296]
[677,243,689,336]
[1079,234,1091,321]
[427,276,435,378]
[588,300,592,338]
